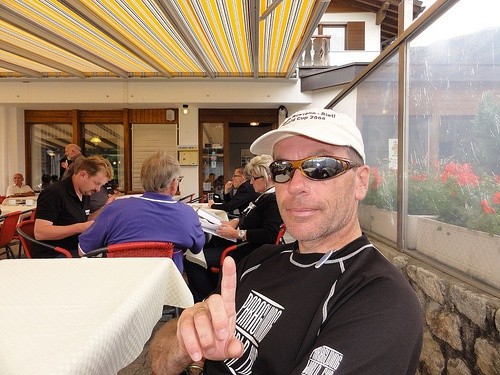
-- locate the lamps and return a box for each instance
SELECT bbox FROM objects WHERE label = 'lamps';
[250,119,260,126]
[91,124,102,145]
[48,149,56,156]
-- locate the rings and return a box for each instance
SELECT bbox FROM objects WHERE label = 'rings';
[193,306,207,313]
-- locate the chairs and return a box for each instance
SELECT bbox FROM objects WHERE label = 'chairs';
[0,186,288,322]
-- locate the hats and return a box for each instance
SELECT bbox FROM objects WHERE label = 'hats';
[250,108,366,164]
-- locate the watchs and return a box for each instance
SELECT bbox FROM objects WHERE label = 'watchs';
[238,229,247,241]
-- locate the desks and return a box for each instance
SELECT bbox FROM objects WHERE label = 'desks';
[0,257,194,375]
[0,195,38,224]
[187,200,223,207]
[201,208,231,242]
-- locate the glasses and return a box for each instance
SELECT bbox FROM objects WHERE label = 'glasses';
[177,176,184,182]
[252,176,264,181]
[269,155,359,184]
[233,175,242,177]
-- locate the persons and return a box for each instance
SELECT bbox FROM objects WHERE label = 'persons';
[36,174,59,191]
[31,155,115,259]
[59,144,86,181]
[5,173,35,197]
[77,151,206,287]
[149,106,425,375]
[183,154,287,298]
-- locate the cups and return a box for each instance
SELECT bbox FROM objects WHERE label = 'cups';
[208,193,214,200]
